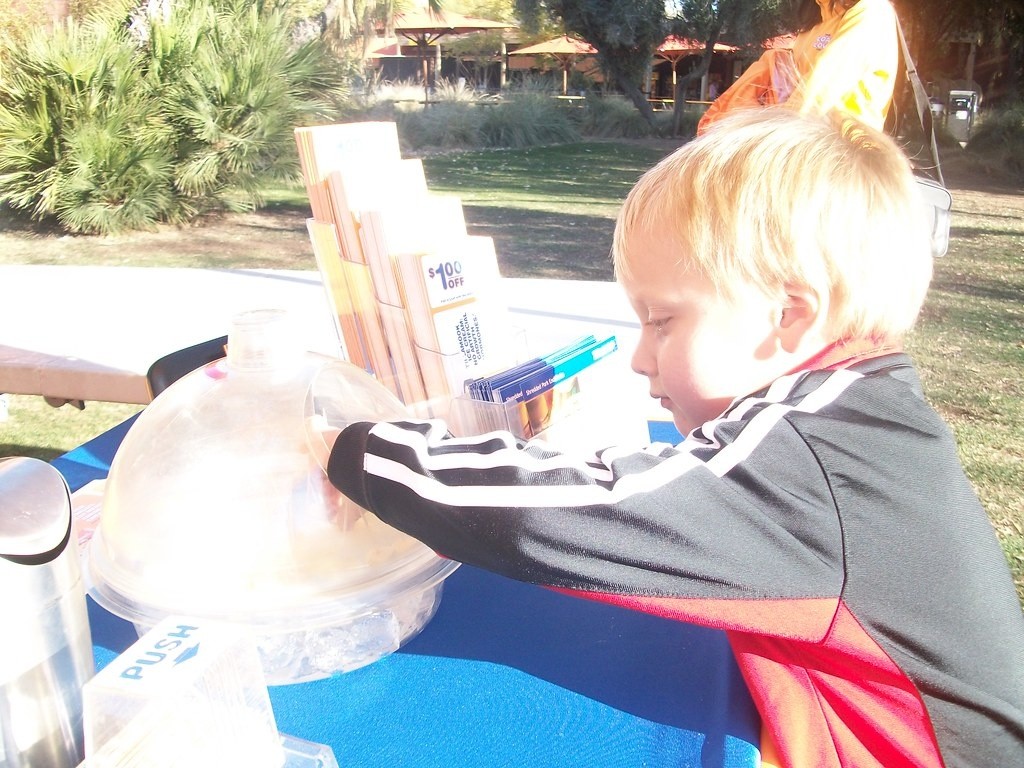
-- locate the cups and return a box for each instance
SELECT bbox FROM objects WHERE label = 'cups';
[0,456,94,768]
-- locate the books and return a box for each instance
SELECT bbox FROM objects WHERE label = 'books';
[292,121,620,442]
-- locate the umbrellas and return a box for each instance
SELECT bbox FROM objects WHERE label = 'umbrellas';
[509,36,599,95]
[728,27,812,51]
[632,33,741,107]
[353,9,517,108]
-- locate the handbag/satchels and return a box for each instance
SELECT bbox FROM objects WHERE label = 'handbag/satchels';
[914,174,952,258]
[696,47,798,138]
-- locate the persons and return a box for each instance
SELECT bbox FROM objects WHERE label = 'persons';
[709,81,720,101]
[287,107,1024,768]
[697,0,899,136]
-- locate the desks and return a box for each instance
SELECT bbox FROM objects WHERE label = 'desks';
[0,399,778,768]
